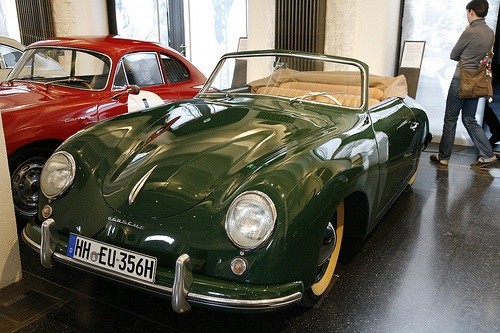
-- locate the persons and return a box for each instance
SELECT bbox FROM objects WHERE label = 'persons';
[484,101,500,145]
[429,0,497,169]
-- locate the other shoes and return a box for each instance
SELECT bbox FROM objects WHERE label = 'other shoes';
[488,134,500,144]
[471,159,497,168]
[430,154,448,165]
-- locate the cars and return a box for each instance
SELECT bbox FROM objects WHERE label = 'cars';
[0,36,219,222]
[19,49,431,314]
[0,35,70,81]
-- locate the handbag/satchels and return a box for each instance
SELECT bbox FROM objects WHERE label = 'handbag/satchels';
[459,62,493,99]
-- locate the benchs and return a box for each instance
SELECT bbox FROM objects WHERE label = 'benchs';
[257,81,382,110]
